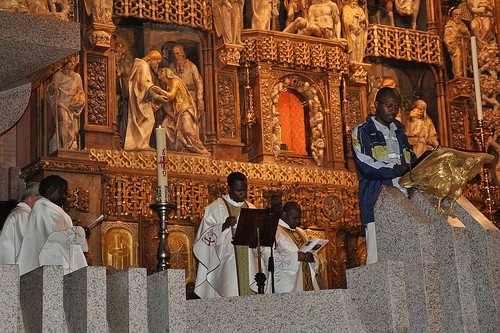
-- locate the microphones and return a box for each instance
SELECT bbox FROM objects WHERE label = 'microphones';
[402,134,412,152]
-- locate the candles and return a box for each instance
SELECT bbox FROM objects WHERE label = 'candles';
[471,36,483,119]
[155,125,167,186]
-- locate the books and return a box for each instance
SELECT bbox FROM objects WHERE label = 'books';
[300,238,330,255]
[85,214,109,231]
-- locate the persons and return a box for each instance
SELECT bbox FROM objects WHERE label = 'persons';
[0,180,41,264]
[46,0,500,158]
[350,86,435,268]
[249,200,321,294]
[192,172,258,300]
[14,174,88,275]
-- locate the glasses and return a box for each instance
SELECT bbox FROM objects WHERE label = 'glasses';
[377,100,402,111]
